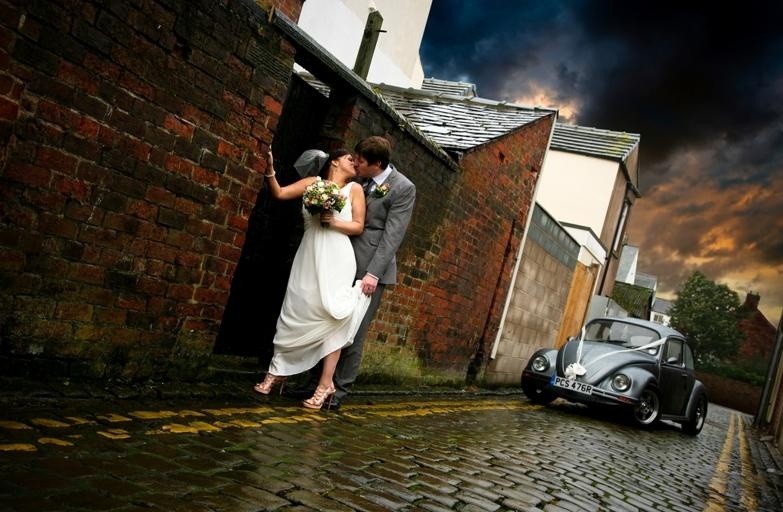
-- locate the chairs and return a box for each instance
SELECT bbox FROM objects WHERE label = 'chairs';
[606,328,631,347]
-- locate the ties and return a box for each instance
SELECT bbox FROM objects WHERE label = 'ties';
[363,179,375,195]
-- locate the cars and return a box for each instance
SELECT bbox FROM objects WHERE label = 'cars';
[521,317,707,436]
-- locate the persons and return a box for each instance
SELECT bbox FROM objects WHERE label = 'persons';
[252,140,373,411]
[280,134,417,411]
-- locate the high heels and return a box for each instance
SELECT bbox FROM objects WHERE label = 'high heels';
[253,373,286,395]
[304,382,336,409]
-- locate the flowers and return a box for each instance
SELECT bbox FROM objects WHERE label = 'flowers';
[300,179,347,228]
[374,182,391,197]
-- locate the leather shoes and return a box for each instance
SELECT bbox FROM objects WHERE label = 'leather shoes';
[326,394,340,409]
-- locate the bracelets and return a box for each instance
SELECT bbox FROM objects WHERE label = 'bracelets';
[263,169,275,177]
[365,271,379,280]
